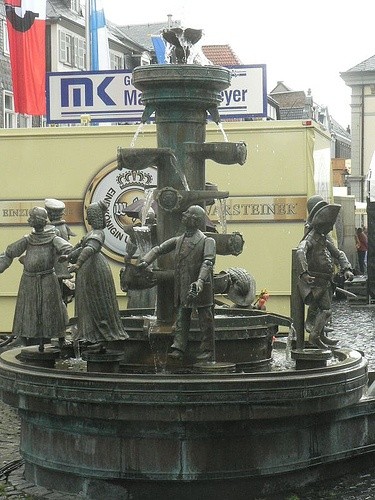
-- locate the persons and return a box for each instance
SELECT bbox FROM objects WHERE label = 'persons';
[135,205,216,366]
[292,195,355,348]
[0,206,76,354]
[355,227,368,274]
[122,204,158,310]
[40,198,76,303]
[55,199,129,355]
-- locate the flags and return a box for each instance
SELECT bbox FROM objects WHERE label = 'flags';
[4,0,48,117]
